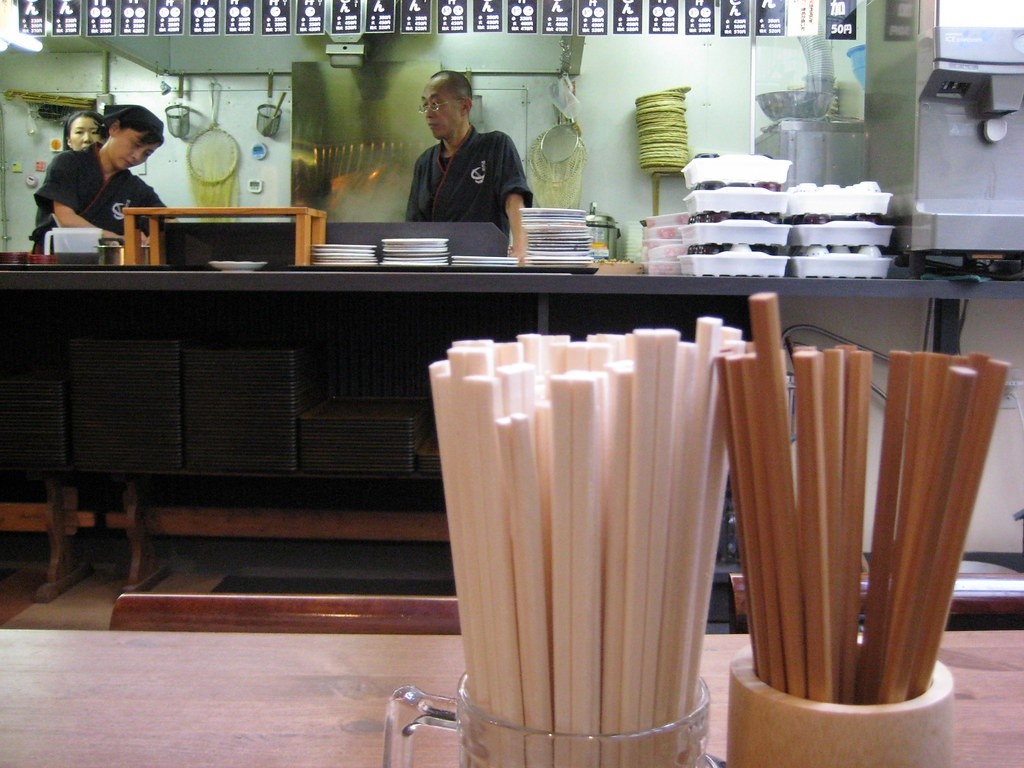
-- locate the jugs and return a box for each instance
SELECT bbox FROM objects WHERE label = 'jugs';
[43,227,103,264]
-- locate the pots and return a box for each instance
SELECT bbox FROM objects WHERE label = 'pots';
[585,202,621,260]
[94,238,151,265]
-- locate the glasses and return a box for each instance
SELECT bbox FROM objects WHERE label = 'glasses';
[417,96,464,113]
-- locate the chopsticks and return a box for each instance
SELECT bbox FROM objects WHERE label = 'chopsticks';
[428,315,749,768]
[717,290,1010,704]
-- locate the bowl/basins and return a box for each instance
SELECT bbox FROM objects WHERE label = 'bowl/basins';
[755,90,836,122]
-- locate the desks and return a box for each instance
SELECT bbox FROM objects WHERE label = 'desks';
[0,630,1024,768]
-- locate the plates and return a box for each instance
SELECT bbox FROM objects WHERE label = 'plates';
[380,238,450,266]
[0,252,55,264]
[208,260,268,272]
[311,244,378,264]
[518,208,594,267]
[451,255,518,267]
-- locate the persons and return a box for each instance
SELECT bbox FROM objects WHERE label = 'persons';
[27,104,173,254]
[405,70,534,262]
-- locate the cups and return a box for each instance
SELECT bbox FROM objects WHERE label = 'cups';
[728,642,957,768]
[381,670,712,768]
